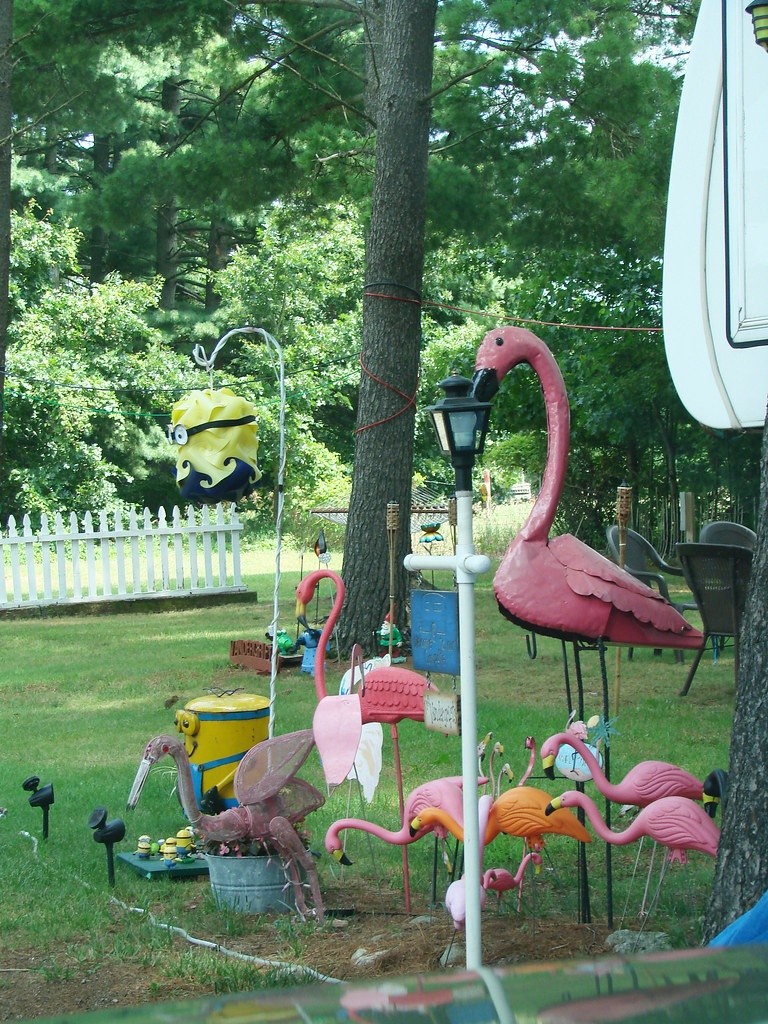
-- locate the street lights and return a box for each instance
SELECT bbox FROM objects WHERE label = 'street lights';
[423,373,495,970]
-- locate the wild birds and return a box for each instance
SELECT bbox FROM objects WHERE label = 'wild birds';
[127,325,721,948]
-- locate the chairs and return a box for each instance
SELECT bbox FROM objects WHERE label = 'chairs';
[603,516,758,698]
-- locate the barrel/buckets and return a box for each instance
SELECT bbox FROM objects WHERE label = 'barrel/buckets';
[205,847,311,915]
